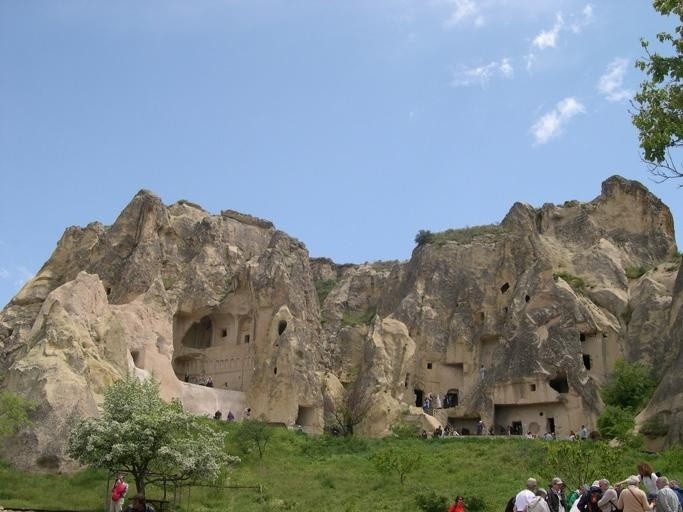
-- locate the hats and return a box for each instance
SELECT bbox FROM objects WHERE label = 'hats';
[592,480,600,487]
[527,478,536,485]
[552,477,563,484]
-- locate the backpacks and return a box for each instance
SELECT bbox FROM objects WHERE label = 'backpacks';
[586,490,602,512]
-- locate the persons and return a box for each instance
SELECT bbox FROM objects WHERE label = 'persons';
[569,431,576,440]
[504,461,683,512]
[205,376,214,386]
[109,479,126,512]
[580,425,588,441]
[241,407,252,420]
[228,412,234,422]
[185,369,189,381]
[447,495,464,512]
[215,411,222,420]
[420,394,556,441]
[120,476,129,509]
[123,493,155,512]
[480,364,486,380]
